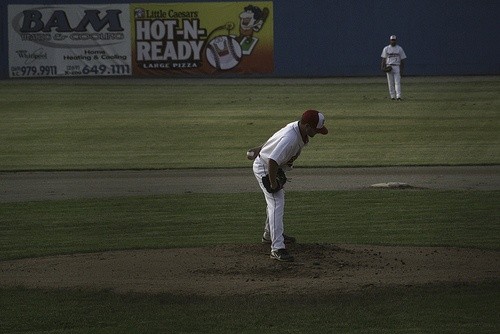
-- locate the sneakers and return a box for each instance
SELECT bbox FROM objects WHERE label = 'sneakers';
[270,248,294,262]
[262,233,295,243]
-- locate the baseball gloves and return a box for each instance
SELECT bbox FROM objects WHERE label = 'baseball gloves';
[382,67,392,72]
[262,168,286,193]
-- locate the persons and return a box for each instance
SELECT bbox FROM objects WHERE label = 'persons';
[251,109,328,261]
[381,35,408,101]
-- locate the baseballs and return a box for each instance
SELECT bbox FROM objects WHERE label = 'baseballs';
[247,151,254,157]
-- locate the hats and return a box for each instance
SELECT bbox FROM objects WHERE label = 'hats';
[301,110,328,135]
[390,35,396,39]
